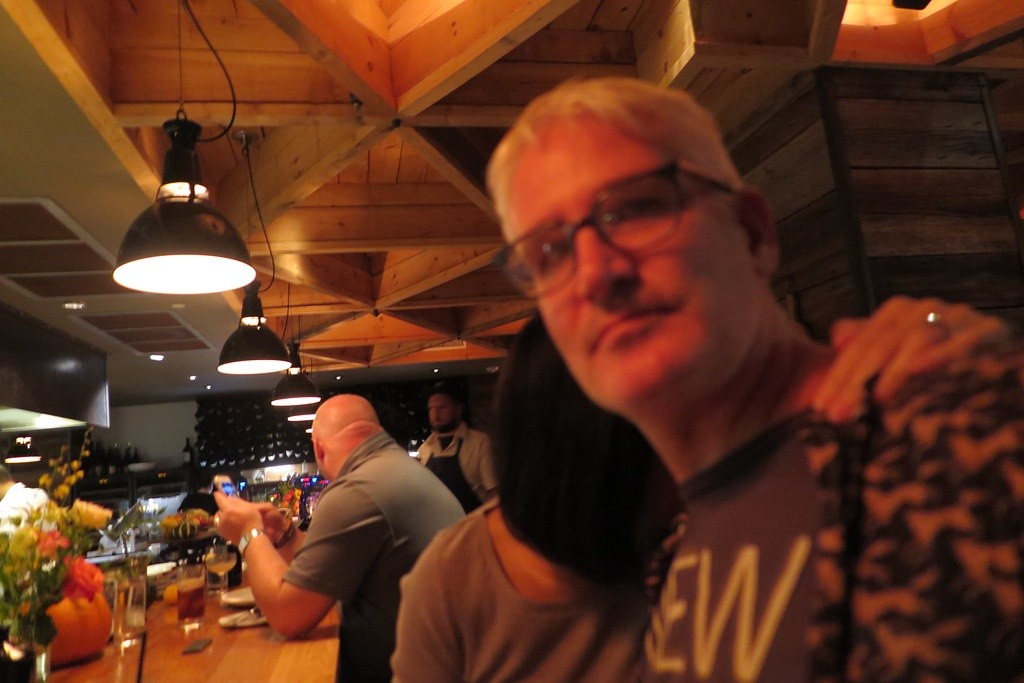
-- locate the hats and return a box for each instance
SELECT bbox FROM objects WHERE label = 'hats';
[429,382,459,403]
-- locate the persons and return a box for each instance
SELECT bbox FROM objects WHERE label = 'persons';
[213,394,466,683]
[415,388,498,512]
[392,320,679,683]
[486,76,1024,683]
[178,473,219,515]
[0,463,57,534]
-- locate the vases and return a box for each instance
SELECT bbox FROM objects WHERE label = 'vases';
[0,626,35,683]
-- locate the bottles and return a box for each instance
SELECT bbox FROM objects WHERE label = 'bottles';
[98,478,110,490]
[157,473,169,484]
[297,477,329,533]
[83,439,139,477]
[183,438,193,468]
[272,494,281,511]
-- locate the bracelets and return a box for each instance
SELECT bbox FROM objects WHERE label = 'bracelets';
[274,510,294,548]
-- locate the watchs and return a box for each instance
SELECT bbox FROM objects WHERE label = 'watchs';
[239,528,264,556]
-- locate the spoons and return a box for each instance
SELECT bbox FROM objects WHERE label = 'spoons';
[231,605,263,625]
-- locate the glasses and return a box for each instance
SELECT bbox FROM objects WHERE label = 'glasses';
[491,164,744,296]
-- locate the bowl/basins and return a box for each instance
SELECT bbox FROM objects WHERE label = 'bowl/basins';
[127,462,156,474]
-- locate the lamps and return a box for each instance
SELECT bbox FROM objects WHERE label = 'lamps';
[286,358,316,421]
[110,1,257,295]
[217,127,292,374]
[268,336,323,406]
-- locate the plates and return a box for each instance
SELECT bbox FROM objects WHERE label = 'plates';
[221,586,256,605]
[218,606,266,627]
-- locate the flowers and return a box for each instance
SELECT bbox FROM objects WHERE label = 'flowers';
[1,427,114,655]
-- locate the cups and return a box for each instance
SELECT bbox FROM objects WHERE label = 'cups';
[112,573,146,647]
[176,564,208,631]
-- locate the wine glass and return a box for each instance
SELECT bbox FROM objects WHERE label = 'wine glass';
[204,546,237,608]
